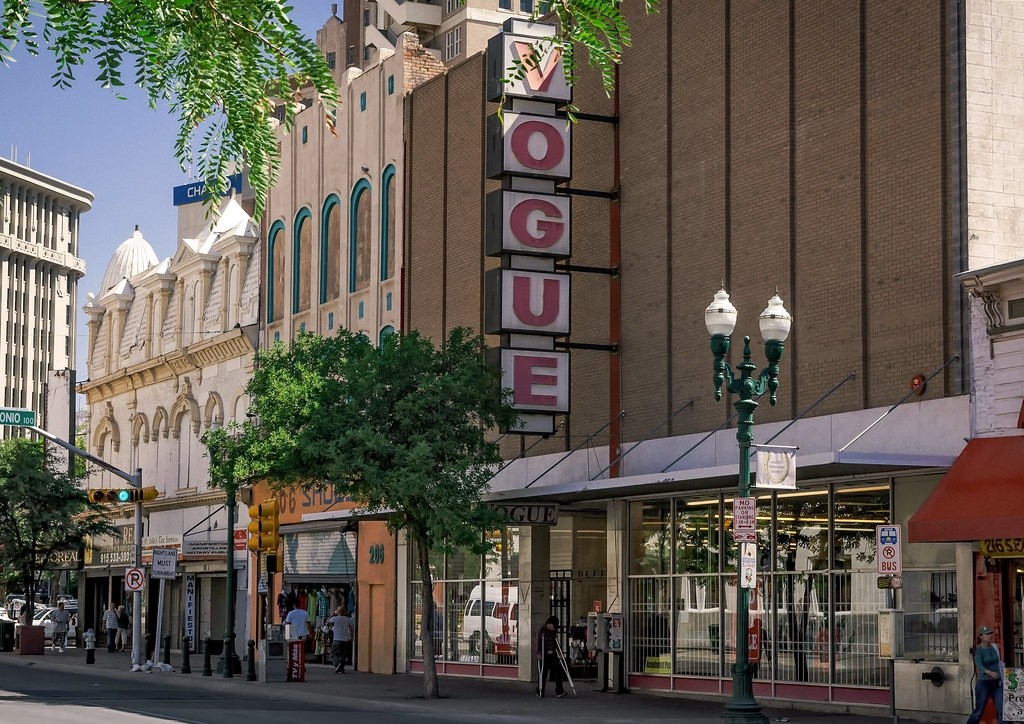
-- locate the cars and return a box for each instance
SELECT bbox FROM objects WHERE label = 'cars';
[0,593,79,640]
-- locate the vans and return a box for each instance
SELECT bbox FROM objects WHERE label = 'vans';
[462,584,520,655]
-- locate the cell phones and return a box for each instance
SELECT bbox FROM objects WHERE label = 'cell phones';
[335,611,339,613]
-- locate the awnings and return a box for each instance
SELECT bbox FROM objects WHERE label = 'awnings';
[907,435,1024,543]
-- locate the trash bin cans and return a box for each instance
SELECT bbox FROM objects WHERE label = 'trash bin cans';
[0,620,15,652]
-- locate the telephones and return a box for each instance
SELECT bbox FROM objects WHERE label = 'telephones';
[593,624,598,636]
[606,624,612,637]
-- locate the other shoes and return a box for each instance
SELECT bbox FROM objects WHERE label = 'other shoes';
[59,648,64,652]
[536,691,544,697]
[556,690,567,698]
[335,663,344,673]
[52,644,55,650]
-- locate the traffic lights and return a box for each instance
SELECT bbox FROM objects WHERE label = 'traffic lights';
[263,553,286,576]
[247,499,280,551]
[89,487,160,503]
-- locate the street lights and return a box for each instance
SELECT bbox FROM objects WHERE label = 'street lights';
[705,280,795,718]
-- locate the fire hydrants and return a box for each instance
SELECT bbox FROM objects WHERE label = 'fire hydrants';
[80,628,97,665]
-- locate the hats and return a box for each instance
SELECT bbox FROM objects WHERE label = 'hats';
[977,625,993,639]
[335,606,346,614]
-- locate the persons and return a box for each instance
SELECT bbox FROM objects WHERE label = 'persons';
[419,602,444,656]
[51,602,70,653]
[534,616,568,699]
[965,626,1004,724]
[282,599,311,673]
[327,607,350,672]
[748,618,771,679]
[102,602,129,653]
[19,604,26,625]
